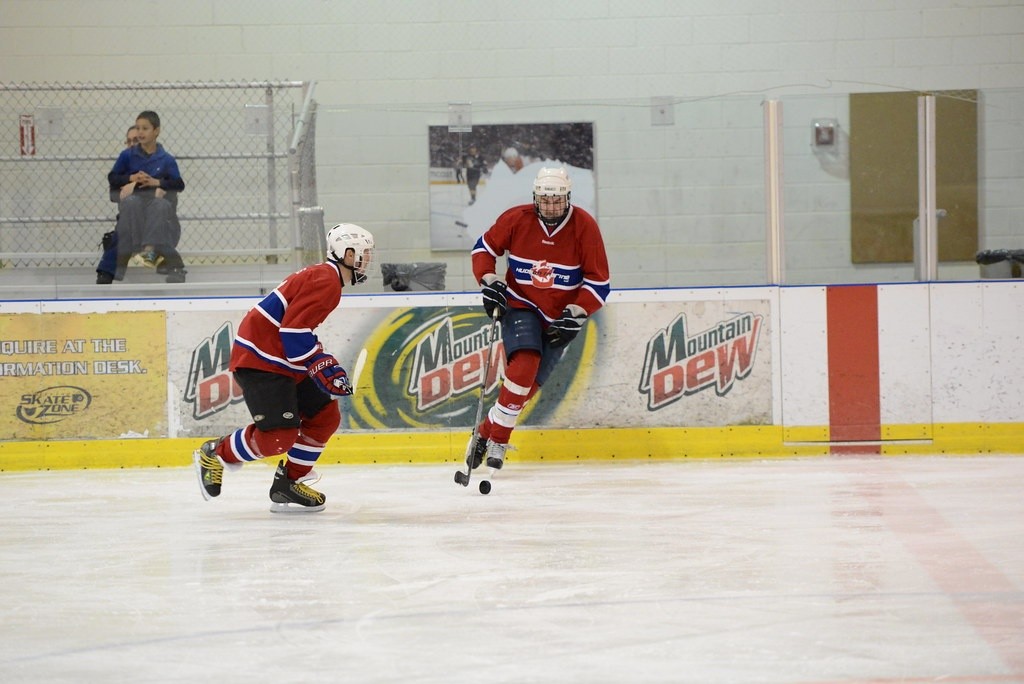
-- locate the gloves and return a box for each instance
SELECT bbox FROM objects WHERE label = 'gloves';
[480,273,507,322]
[547,304,588,349]
[482,170,488,173]
[307,355,354,397]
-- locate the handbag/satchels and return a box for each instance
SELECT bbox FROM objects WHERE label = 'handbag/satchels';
[96,232,118,284]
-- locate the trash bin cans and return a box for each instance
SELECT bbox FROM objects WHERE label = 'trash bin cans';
[379,259,445,292]
[976,247,1024,280]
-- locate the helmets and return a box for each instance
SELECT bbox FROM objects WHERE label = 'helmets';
[469,143,478,147]
[325,223,375,287]
[533,167,572,224]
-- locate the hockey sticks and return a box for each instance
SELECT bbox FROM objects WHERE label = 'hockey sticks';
[349,348,368,395]
[454,306,498,487]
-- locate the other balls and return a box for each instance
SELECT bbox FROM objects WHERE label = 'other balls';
[478,481,491,494]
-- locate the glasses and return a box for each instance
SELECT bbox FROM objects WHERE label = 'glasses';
[123,139,139,144]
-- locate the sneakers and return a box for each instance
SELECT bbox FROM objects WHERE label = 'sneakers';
[465,426,518,479]
[468,199,476,205]
[194,438,223,502]
[156,253,175,274]
[269,459,326,513]
[133,248,159,269]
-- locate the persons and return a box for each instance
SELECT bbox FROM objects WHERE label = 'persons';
[96,111,185,284]
[455,143,489,205]
[193,223,375,512]
[464,167,610,477]
